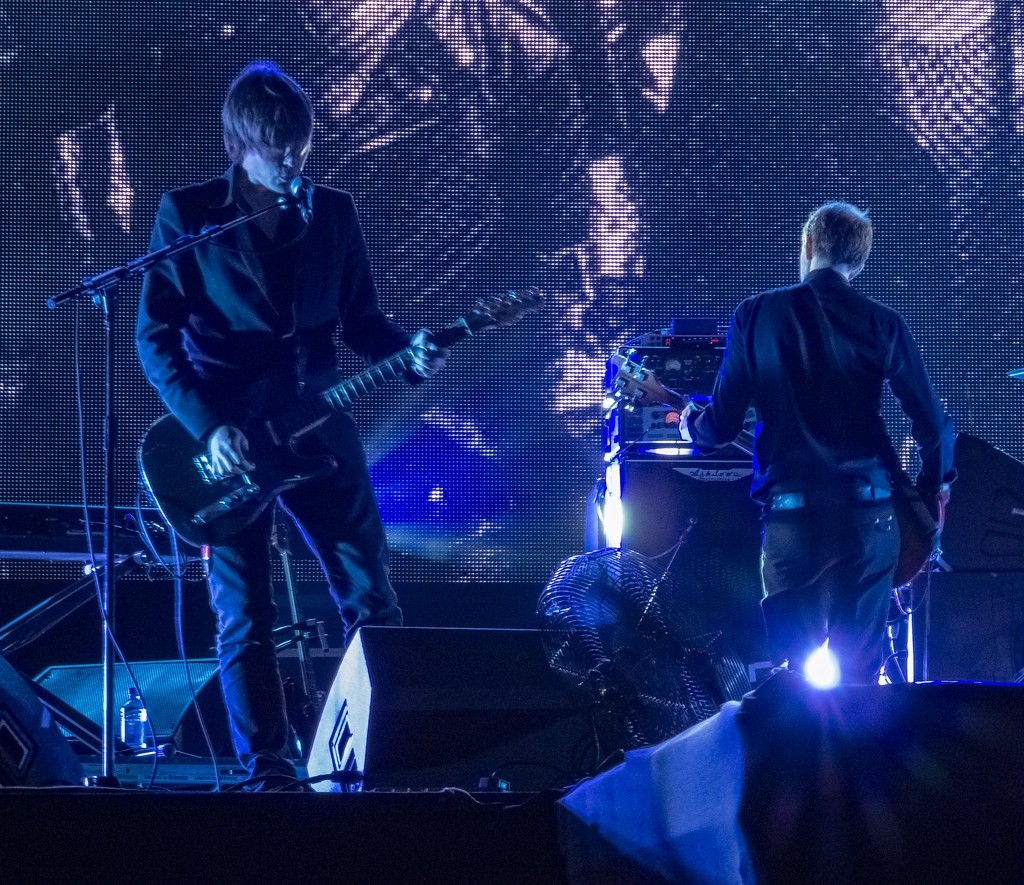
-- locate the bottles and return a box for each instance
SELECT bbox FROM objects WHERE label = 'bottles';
[120,688,147,750]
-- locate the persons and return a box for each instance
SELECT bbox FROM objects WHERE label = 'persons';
[131,66,454,790]
[676,202,960,676]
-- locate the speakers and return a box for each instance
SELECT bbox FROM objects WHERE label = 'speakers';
[308,624,600,791]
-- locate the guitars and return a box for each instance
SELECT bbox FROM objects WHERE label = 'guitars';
[137,287,546,547]
[611,349,946,591]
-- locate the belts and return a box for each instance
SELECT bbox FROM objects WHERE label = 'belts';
[772,483,896,514]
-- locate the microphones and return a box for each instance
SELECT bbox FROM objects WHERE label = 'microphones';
[290,175,315,224]
